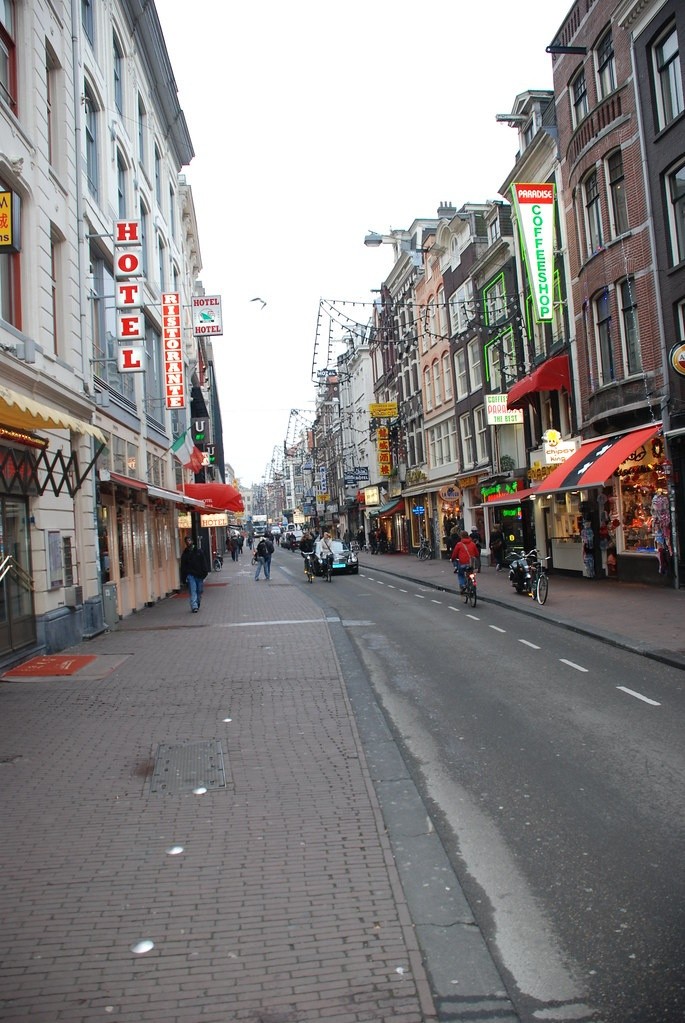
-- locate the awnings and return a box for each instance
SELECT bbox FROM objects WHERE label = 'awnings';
[507,355,572,411]
[369,497,405,519]
[533,426,663,496]
[99,469,205,513]
[0,385,110,498]
[175,484,245,515]
[467,504,484,510]
[480,487,537,507]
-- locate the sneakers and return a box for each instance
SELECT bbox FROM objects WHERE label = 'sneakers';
[460,586,466,594]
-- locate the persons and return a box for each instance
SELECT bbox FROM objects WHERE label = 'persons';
[489,524,505,572]
[446,512,464,537]
[180,536,209,613]
[448,525,482,573]
[299,532,317,574]
[451,530,479,593]
[275,534,280,544]
[319,532,334,572]
[226,533,253,561]
[254,530,275,581]
[289,533,296,547]
[343,525,387,555]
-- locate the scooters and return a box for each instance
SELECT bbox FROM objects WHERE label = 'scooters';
[505,549,538,594]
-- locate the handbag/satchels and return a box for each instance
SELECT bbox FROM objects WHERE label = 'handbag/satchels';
[470,557,481,569]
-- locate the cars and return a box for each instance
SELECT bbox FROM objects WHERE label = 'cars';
[270,526,281,535]
[281,531,305,548]
[312,541,360,575]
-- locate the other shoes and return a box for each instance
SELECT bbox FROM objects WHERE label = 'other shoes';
[454,567,457,573]
[496,564,500,571]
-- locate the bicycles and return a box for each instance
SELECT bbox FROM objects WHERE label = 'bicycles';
[320,554,332,583]
[252,548,258,565]
[417,536,432,560]
[452,559,477,608]
[290,540,296,553]
[302,550,315,584]
[529,554,552,605]
[213,551,222,572]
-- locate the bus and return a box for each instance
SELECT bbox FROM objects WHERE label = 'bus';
[281,524,296,532]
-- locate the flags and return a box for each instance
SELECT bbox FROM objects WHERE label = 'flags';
[170,430,204,474]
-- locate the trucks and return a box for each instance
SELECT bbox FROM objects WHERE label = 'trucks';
[252,515,268,537]
